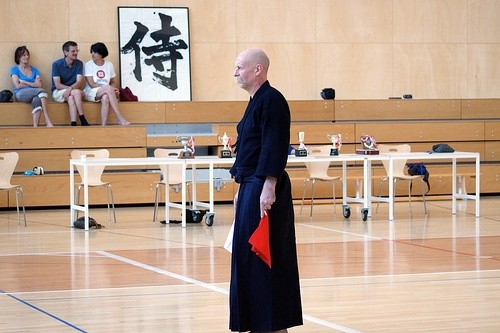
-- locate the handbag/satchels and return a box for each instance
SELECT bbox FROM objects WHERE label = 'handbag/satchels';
[432,144,455,152]
[119,86,138,102]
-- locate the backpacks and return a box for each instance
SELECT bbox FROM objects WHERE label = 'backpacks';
[0,90,14,103]
[406,162,430,180]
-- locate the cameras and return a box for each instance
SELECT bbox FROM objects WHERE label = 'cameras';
[321,88,335,100]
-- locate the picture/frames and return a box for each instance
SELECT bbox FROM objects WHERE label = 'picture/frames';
[118,6,192,102]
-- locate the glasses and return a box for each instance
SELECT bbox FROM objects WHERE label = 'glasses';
[69,49,81,52]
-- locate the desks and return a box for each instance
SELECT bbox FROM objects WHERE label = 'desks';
[67,152,481,231]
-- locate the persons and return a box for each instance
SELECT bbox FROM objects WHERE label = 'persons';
[10,46,54,127]
[230,47,303,333]
[83,42,132,126]
[51,41,90,126]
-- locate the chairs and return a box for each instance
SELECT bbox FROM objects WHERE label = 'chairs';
[375,143,429,218]
[70,148,116,226]
[299,145,341,219]
[0,152,28,225]
[153,147,192,221]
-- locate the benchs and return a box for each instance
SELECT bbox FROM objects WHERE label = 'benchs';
[0,98,500,207]
